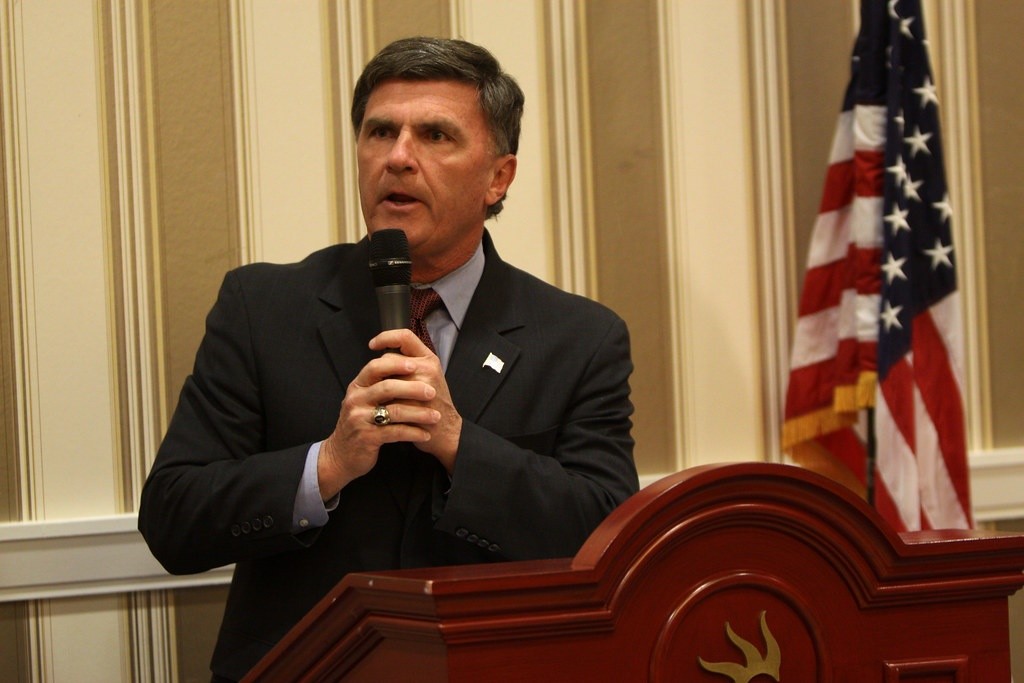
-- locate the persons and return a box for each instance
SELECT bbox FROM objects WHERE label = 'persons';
[138,37,640,683]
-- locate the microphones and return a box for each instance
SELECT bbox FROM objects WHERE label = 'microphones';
[367,228,411,380]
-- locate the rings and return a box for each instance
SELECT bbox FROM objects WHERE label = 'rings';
[374,406,390,425]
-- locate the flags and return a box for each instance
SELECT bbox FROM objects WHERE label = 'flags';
[781,0,972,535]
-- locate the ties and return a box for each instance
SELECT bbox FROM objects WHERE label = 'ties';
[407,286,444,358]
